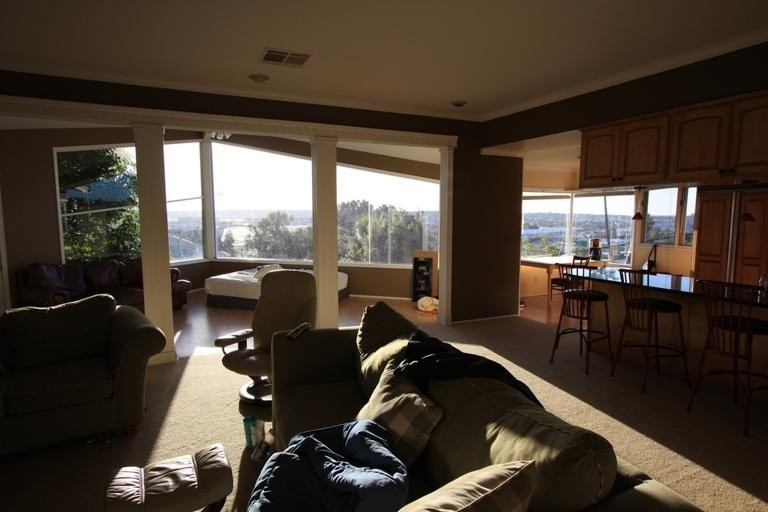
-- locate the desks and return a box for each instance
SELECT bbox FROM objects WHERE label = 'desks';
[521,256,608,313]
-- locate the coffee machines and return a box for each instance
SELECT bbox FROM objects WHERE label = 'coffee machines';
[588,238,602,262]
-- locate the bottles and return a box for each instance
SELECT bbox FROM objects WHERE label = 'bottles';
[758,275,766,288]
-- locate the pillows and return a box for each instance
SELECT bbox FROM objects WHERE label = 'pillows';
[353,359,443,471]
[394,462,536,511]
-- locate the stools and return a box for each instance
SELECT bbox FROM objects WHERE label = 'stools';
[103,448,234,512]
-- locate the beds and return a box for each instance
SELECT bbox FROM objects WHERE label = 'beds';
[204,267,350,311]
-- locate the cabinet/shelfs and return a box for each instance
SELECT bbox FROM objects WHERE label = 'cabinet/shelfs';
[578,88,768,288]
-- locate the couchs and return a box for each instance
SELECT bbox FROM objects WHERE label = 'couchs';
[271,327,704,512]
[16,255,191,309]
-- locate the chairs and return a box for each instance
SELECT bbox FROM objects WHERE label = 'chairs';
[685,280,768,437]
[550,263,614,374]
[215,269,317,408]
[550,256,591,309]
[0,292,167,457]
[610,270,693,394]
